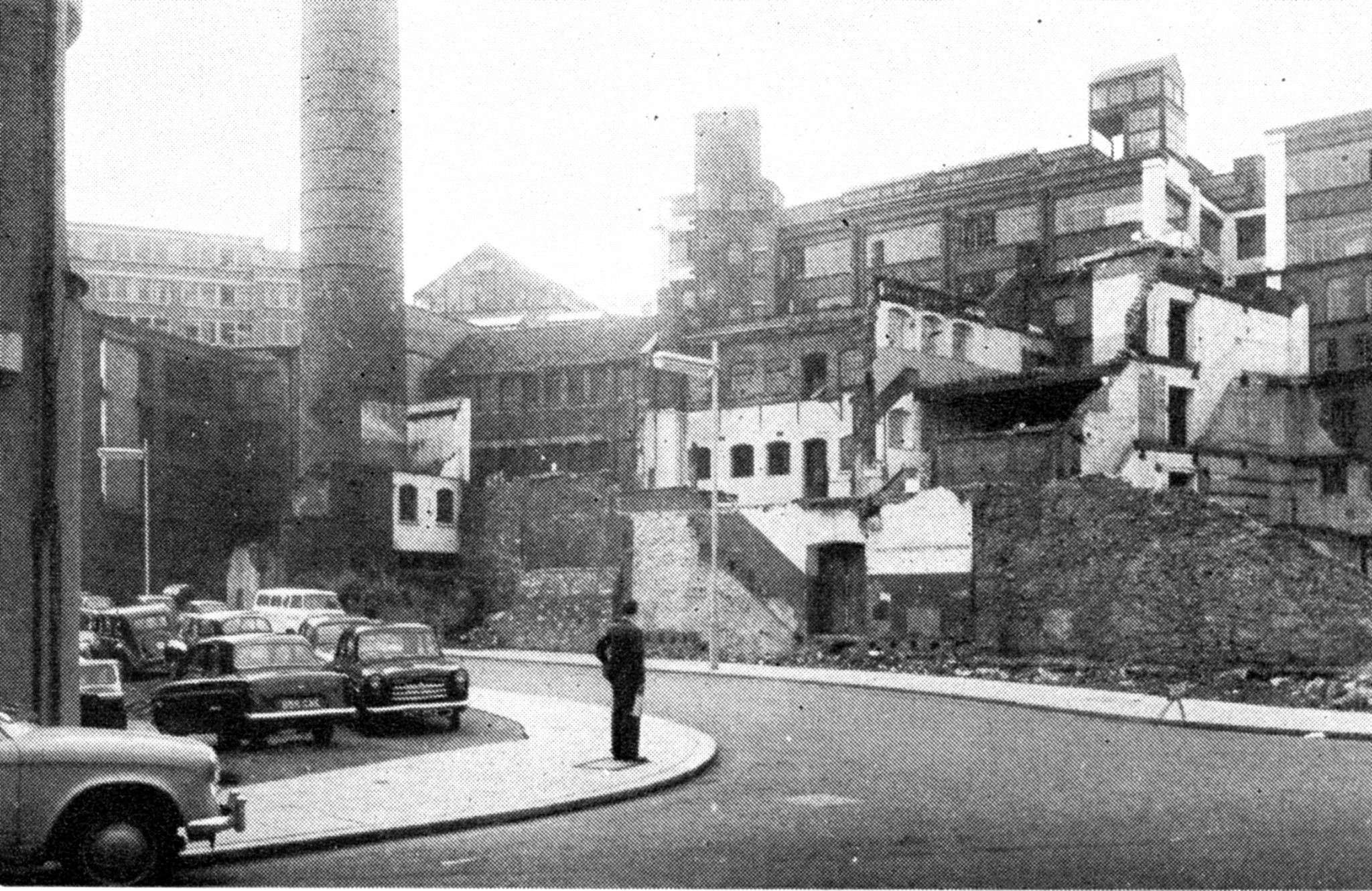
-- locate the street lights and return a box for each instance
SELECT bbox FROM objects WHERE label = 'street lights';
[653,343,722,672]
[97,438,151,593]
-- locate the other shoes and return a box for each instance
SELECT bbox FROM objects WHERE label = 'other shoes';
[610,752,649,764]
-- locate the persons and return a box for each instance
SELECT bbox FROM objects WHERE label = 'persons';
[596,597,649,763]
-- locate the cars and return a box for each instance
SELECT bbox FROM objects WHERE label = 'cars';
[0,706,248,887]
[149,632,359,752]
[74,580,386,730]
[327,618,472,739]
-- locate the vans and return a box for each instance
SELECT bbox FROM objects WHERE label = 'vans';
[248,587,345,635]
